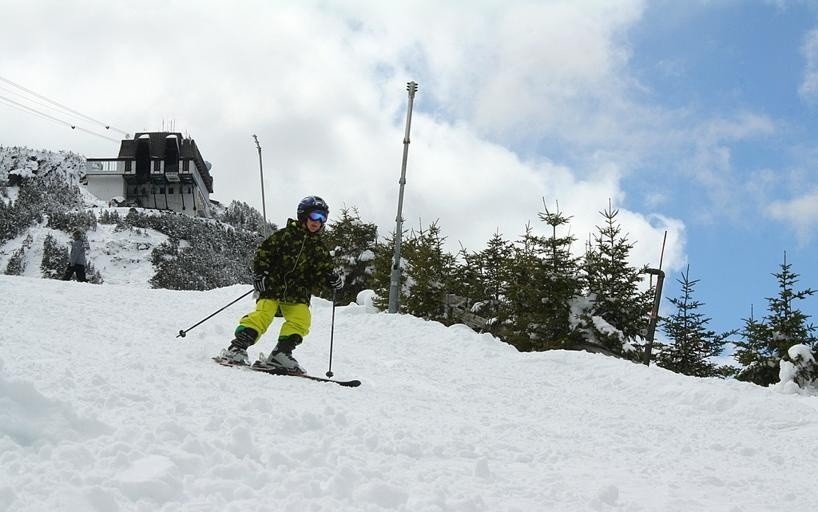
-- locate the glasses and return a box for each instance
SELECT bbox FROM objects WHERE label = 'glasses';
[303,205,328,223]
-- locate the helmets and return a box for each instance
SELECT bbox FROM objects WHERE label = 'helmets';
[297,196,329,223]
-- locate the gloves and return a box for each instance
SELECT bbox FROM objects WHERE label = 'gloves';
[253,271,269,292]
[327,273,342,290]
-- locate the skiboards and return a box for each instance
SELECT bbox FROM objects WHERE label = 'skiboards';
[212,358,361,387]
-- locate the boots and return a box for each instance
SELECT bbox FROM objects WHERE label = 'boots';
[265,333,306,372]
[224,328,258,362]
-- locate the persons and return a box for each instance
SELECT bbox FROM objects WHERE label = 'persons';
[59,231,86,282]
[223,196,343,375]
